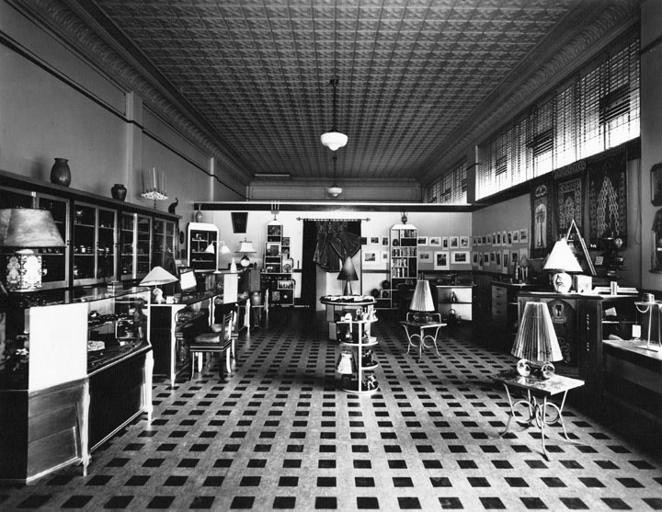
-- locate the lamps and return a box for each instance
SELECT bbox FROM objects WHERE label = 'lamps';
[327,155,343,197]
[543,240,584,293]
[320,78,349,153]
[0,207,67,289]
[234,237,257,267]
[510,300,564,379]
[137,264,180,305]
[336,254,359,297]
[409,272,436,323]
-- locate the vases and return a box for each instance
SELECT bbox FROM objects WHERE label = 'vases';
[196,204,203,222]
[49,158,71,186]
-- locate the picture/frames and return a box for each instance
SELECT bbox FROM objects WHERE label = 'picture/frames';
[360,236,389,262]
[472,228,528,274]
[417,236,471,272]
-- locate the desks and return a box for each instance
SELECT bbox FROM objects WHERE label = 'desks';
[485,372,585,462]
[320,297,379,343]
[399,319,448,360]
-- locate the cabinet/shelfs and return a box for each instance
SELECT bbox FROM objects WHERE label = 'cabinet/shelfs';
[260,224,297,308]
[186,222,220,275]
[330,306,382,396]
[391,224,418,309]
[602,338,662,445]
[488,278,535,330]
[0,172,182,348]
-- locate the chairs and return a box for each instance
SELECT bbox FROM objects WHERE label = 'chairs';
[189,302,241,381]
